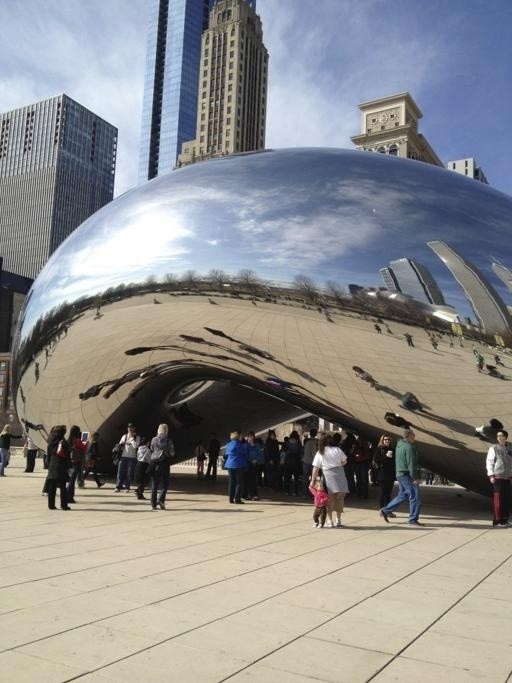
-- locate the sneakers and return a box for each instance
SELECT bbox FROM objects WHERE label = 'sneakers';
[125,488,129,493]
[24,469,34,472]
[336,518,342,527]
[380,509,389,524]
[97,482,106,488]
[229,495,260,504]
[380,511,397,518]
[152,505,157,510]
[48,498,76,510]
[502,521,512,527]
[408,521,425,527]
[0,473,8,477]
[157,501,165,509]
[493,523,509,528]
[324,520,335,528]
[137,496,146,500]
[112,488,121,493]
[317,524,324,528]
[312,523,318,528]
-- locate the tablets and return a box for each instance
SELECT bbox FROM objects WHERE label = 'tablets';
[82,432,89,443]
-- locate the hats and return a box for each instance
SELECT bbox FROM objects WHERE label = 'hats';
[126,422,136,427]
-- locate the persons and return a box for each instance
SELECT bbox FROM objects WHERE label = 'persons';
[18,414,44,436]
[223,429,421,528]
[353,315,512,426]
[43,425,105,510]
[0,424,13,476]
[424,472,448,486]
[487,429,511,526]
[76,327,272,400]
[25,438,39,472]
[115,423,173,509]
[197,437,220,476]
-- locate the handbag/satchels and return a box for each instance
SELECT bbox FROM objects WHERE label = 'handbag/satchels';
[68,440,84,464]
[150,440,172,464]
[56,439,68,459]
[197,446,207,461]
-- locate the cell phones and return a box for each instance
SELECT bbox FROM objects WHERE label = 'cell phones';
[388,451,392,455]
